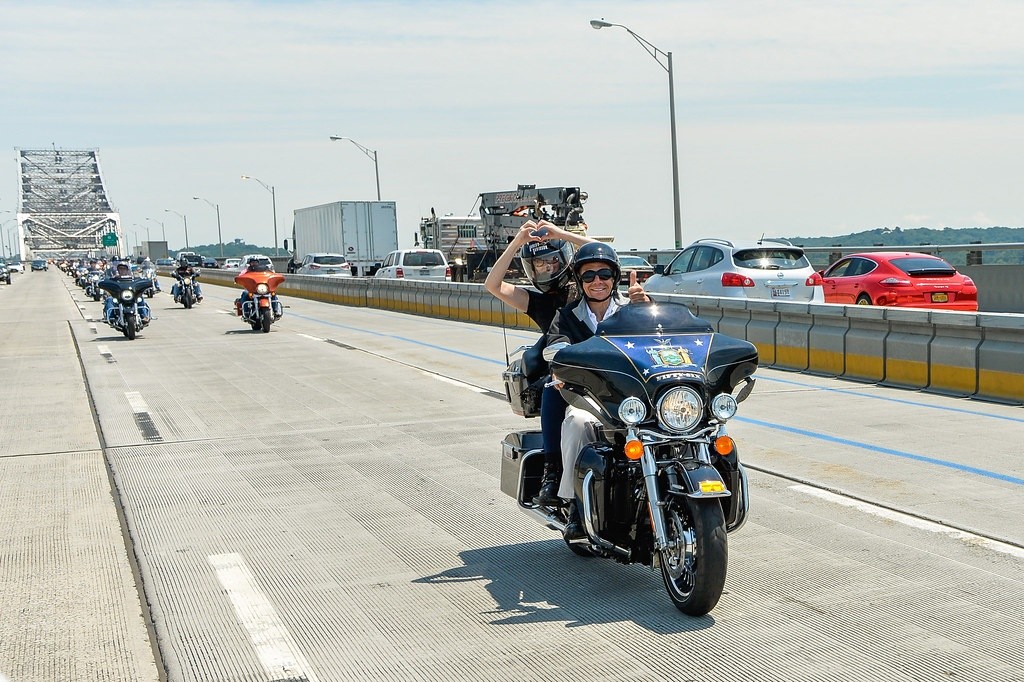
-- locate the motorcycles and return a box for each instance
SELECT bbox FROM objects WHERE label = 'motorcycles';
[54,260,160,340]
[171,265,202,308]
[233,264,289,333]
[500,301,761,618]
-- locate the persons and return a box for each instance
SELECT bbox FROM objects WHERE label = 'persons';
[56,254,282,331]
[485,220,656,540]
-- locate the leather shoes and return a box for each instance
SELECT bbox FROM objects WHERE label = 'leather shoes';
[539,462,566,506]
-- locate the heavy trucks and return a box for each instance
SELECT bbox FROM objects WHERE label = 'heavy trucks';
[415,183,616,283]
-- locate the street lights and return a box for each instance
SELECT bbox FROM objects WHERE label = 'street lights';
[165,209,189,251]
[240,176,279,256]
[0,218,17,257]
[132,224,149,241]
[590,20,683,251]
[193,197,222,258]
[145,217,164,241]
[329,135,380,200]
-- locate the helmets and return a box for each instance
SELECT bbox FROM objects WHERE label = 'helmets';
[572,242,622,290]
[90,256,106,264]
[180,258,189,266]
[520,227,574,293]
[110,255,130,270]
[247,256,260,263]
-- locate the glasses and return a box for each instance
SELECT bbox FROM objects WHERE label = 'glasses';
[577,268,614,283]
[531,255,559,268]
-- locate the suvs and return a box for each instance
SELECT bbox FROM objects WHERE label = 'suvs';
[295,253,352,277]
[641,238,825,301]
[374,249,452,283]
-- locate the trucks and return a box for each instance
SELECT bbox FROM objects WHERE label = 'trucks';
[284,200,399,277]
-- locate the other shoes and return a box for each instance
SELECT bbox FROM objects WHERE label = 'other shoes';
[143,316,150,326]
[564,502,586,540]
[197,295,203,301]
[274,314,281,320]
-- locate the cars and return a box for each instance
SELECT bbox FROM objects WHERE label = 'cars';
[123,241,275,272]
[821,252,979,315]
[618,255,655,291]
[0,256,48,285]
